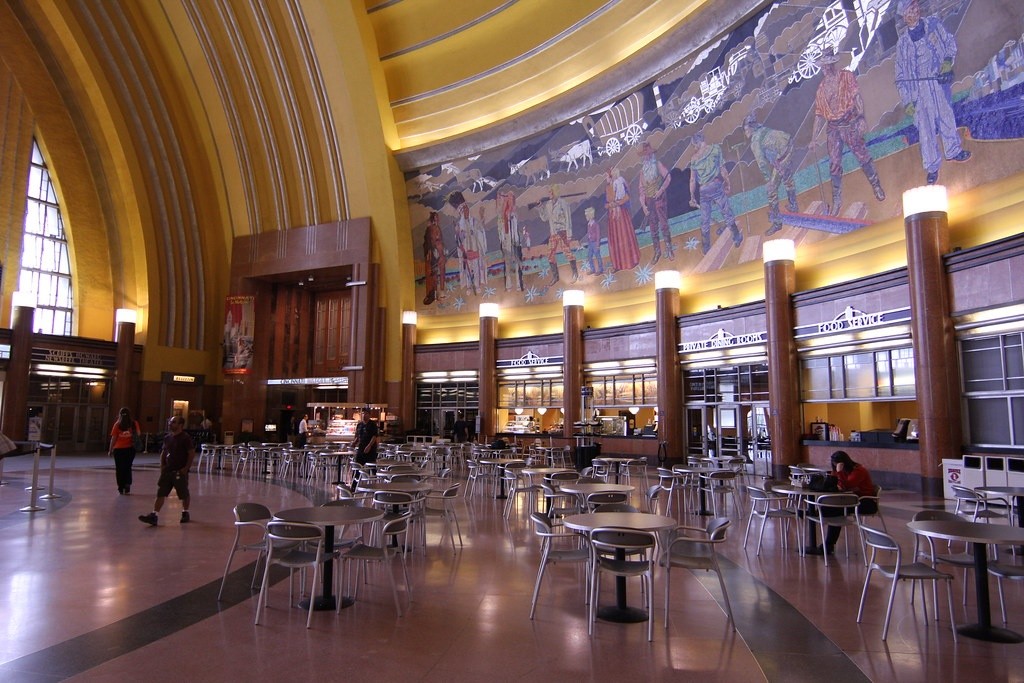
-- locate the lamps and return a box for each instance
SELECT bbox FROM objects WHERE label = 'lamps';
[513,409,526,415]
[629,406,640,414]
[536,407,547,415]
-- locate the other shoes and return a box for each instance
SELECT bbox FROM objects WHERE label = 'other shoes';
[139,513,158,525]
[819,543,834,552]
[120,492,123,494]
[180,512,189,523]
[125,485,130,493]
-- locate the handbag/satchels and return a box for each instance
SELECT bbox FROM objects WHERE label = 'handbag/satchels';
[131,421,144,452]
[808,474,839,492]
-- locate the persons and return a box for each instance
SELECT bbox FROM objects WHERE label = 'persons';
[138,415,195,526]
[350,408,378,494]
[451,413,470,443]
[108,406,141,494]
[297,414,308,448]
[490,436,510,458]
[199,415,213,442]
[818,450,879,553]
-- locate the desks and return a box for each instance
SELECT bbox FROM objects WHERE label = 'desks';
[907,520,1024,644]
[973,486,1024,554]
[250,445,281,475]
[674,467,730,516]
[699,456,744,505]
[536,446,568,467]
[356,483,433,553]
[521,467,576,518]
[560,483,636,508]
[563,512,677,623]
[319,450,354,485]
[273,506,384,611]
[771,485,854,555]
[376,470,432,481]
[205,445,235,472]
[591,457,650,483]
[479,459,525,501]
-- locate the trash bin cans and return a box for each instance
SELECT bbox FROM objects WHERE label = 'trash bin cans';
[1005,457,1024,507]
[573,432,602,472]
[224,431,234,445]
[962,455,984,502]
[313,430,326,443]
[407,435,440,445]
[984,456,1006,505]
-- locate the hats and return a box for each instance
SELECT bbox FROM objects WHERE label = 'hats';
[362,408,371,414]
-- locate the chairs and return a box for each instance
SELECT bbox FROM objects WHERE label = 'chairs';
[194,439,1024,683]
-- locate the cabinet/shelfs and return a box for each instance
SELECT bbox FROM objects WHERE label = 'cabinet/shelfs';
[501,420,533,434]
